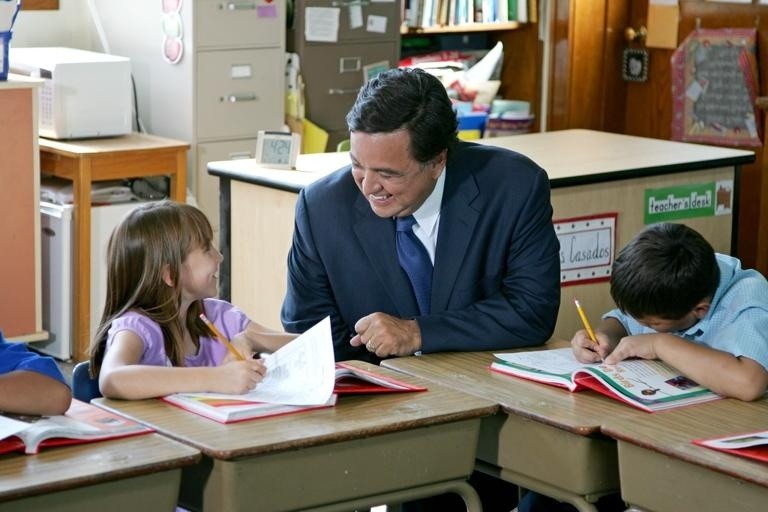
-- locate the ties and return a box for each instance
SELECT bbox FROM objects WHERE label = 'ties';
[394,216,434,316]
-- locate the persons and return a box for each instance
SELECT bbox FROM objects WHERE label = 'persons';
[280,66,561,367]
[87,200,304,402]
[0,333,72,417]
[570,221,768,402]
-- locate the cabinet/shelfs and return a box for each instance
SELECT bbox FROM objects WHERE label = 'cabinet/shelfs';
[285,1,402,152]
[148,1,286,300]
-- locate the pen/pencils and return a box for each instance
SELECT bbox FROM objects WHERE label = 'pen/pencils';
[199,313,264,384]
[573,298,605,363]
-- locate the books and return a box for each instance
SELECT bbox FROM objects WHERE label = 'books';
[692,430,768,463]
[407,0,529,29]
[163,315,427,425]
[0,396,157,456]
[486,348,728,413]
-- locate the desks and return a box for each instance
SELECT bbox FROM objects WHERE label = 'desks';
[36,134,192,367]
[0,335,768,511]
